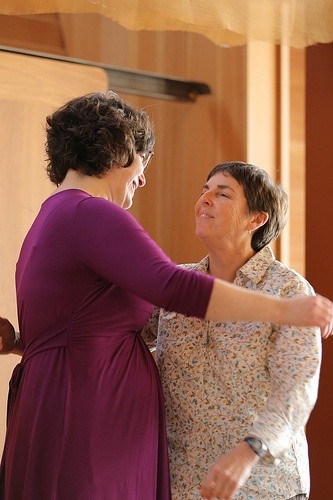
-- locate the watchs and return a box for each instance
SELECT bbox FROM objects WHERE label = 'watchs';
[243,436,266,457]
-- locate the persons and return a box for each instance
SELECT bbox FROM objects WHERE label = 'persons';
[0,161,323,500]
[0,91,333,500]
